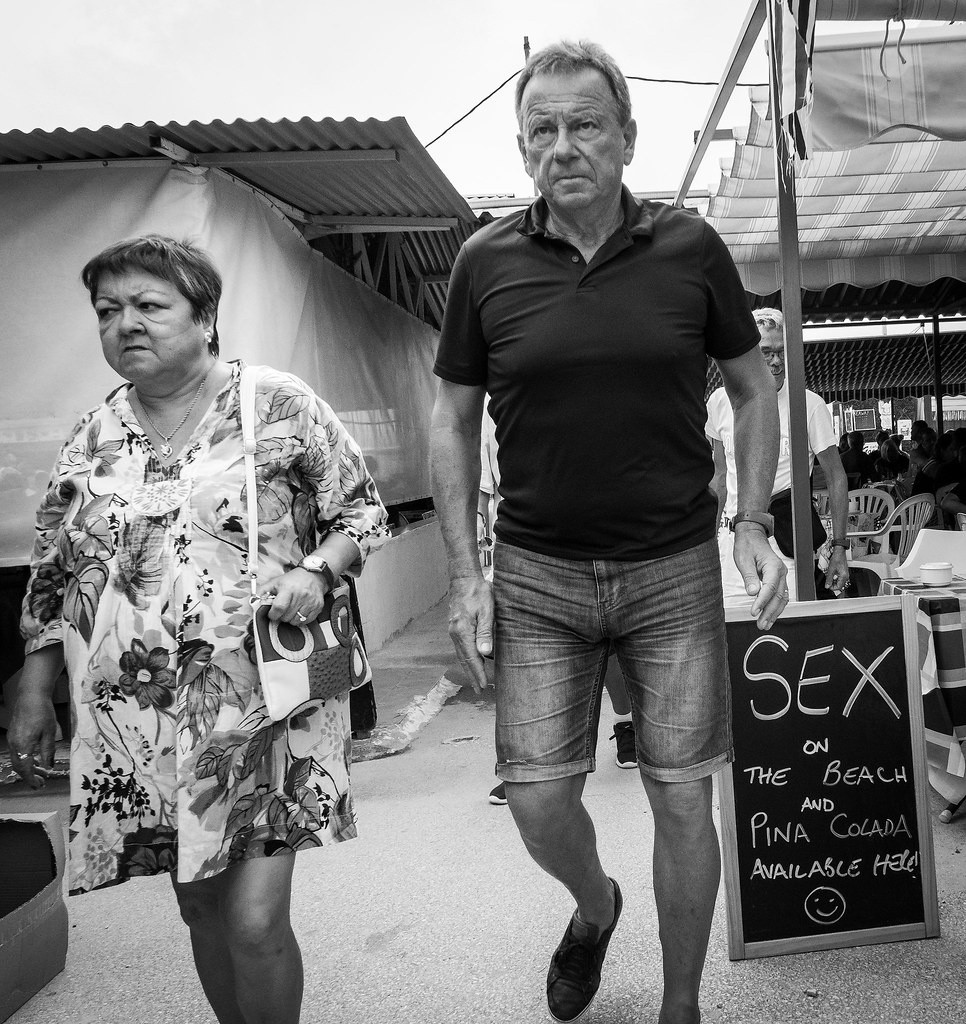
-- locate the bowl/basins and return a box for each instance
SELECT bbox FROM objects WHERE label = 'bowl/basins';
[921,562,952,587]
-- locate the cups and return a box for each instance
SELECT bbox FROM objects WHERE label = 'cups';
[849,502,859,512]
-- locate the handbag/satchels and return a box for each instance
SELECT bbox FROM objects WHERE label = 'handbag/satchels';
[249,577,372,722]
[767,488,828,560]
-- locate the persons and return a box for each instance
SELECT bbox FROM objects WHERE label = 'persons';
[5,234,393,1024]
[813,420,966,555]
[704,307,850,607]
[429,44,792,1024]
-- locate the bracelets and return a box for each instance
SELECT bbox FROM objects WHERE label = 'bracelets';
[477,512,486,527]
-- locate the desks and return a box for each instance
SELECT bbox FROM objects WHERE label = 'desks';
[877,571,966,778]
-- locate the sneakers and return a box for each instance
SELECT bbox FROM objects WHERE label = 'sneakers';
[488,782,507,804]
[609,721,638,768]
[547,875,623,1023]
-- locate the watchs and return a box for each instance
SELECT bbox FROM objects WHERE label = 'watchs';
[729,511,775,538]
[298,554,334,594]
[830,539,850,550]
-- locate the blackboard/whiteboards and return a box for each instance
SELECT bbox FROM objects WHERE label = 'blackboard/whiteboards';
[718,592,943,963]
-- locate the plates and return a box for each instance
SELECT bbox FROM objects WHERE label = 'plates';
[848,510,862,514]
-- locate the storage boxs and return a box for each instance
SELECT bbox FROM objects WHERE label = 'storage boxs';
[0,810,71,1023]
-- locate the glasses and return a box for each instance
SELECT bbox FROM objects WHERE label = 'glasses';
[761,350,784,361]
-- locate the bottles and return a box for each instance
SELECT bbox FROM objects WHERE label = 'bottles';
[898,471,902,482]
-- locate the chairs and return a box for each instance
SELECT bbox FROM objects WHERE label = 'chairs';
[847,489,935,599]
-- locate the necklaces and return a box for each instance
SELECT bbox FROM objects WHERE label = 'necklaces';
[135,359,217,460]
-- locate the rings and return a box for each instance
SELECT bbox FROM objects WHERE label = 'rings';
[17,753,29,760]
[297,611,307,622]
[844,581,852,589]
[785,590,788,592]
